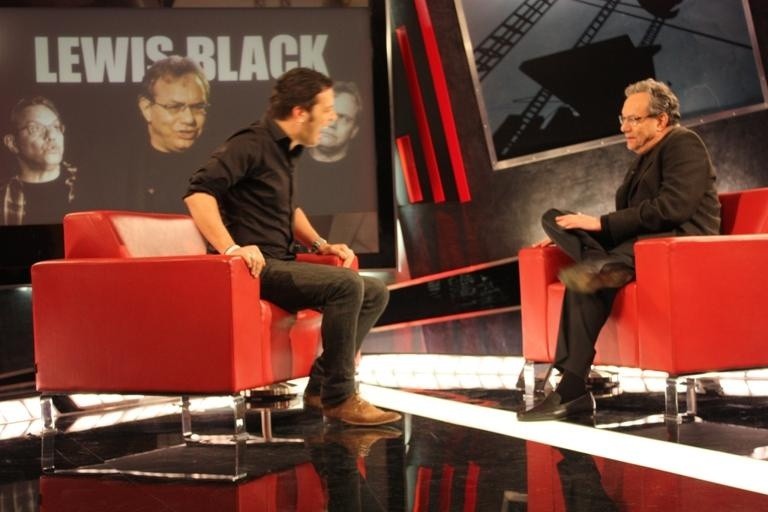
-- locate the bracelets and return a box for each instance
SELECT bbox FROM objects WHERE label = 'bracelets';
[312,238,327,250]
[221,244,242,256]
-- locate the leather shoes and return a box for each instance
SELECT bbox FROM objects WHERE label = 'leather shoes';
[558,259,634,294]
[321,394,402,427]
[302,389,324,415]
[518,391,596,421]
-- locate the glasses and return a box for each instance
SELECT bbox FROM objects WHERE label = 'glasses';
[618,113,658,125]
[16,120,66,135]
[153,100,212,114]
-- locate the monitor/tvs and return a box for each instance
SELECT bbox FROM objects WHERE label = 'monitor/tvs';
[0,0,398,289]
[452,0,768,172]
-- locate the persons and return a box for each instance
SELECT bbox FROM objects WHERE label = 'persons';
[309,431,402,511]
[108,53,222,212]
[180,64,404,429]
[552,448,619,511]
[515,78,730,422]
[0,94,81,227]
[305,78,364,214]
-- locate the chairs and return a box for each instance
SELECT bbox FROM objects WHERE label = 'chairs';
[517,186,768,426]
[29,211,359,482]
